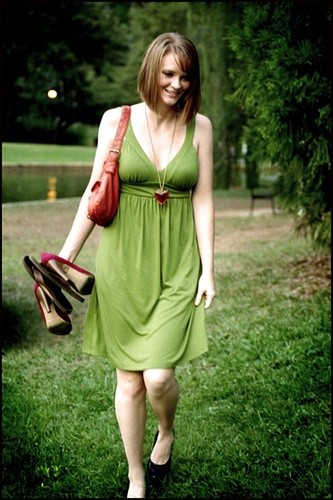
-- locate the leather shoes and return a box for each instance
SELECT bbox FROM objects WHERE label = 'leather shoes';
[147,428,175,480]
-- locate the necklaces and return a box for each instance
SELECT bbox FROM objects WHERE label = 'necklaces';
[144,103,179,206]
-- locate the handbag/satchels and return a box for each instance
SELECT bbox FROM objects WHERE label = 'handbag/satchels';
[88,106,132,228]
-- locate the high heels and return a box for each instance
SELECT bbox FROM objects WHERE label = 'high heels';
[40,253,95,295]
[33,283,72,335]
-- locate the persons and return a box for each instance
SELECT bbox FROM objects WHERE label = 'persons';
[55,32,217,500]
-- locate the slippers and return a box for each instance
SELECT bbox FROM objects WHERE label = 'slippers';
[23,256,85,314]
